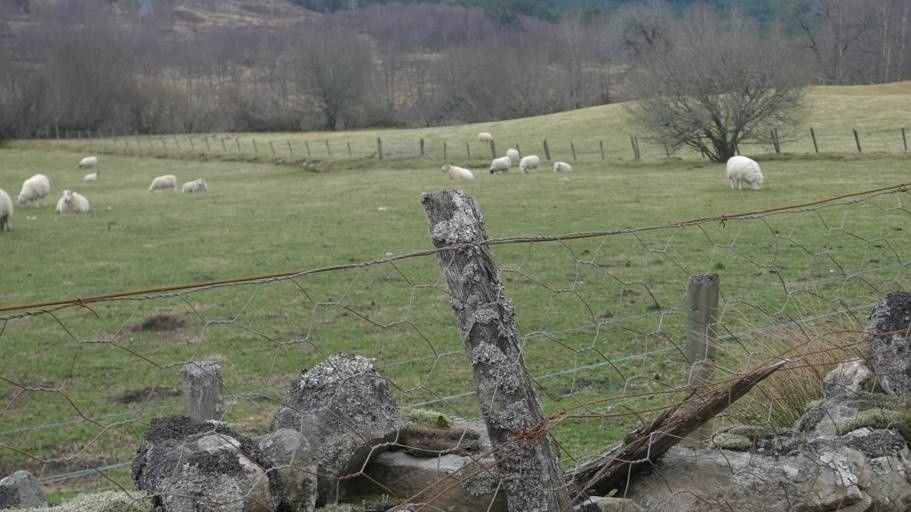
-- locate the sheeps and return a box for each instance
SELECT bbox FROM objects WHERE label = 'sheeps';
[148,174,178,195]
[441,161,475,181]
[1,187,14,232]
[182,178,208,193]
[17,173,51,208]
[726,155,765,192]
[78,156,98,170]
[478,132,494,143]
[55,188,91,214]
[489,149,540,175]
[555,161,575,175]
[82,170,100,184]
